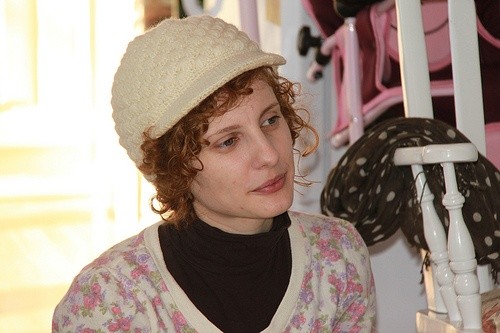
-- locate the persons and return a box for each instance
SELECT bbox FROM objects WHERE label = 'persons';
[52,16,380,333]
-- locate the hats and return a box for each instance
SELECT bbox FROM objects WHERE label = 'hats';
[111,15,287,167]
